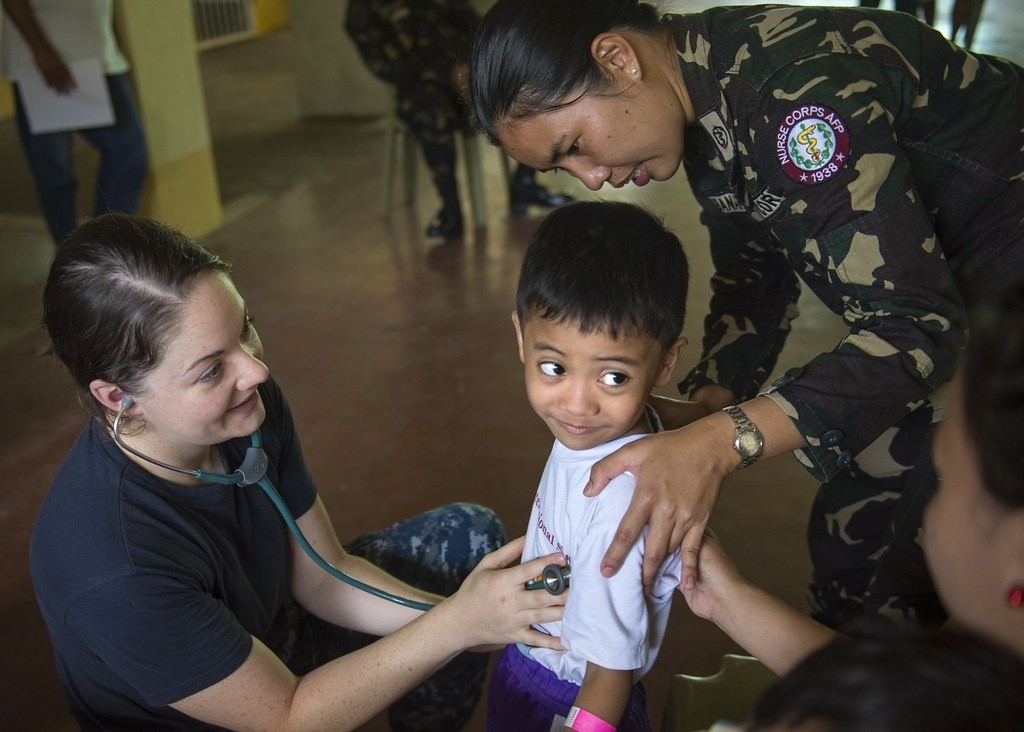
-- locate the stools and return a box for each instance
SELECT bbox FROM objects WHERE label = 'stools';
[373,117,528,228]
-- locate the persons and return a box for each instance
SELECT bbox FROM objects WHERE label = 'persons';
[0,0,150,249]
[676,283,1024,732]
[859,0,985,51]
[343,0,576,241]
[470,0,1024,633]
[27,214,568,732]
[487,199,683,732]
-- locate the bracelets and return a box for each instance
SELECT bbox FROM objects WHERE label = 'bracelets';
[563,705,617,732]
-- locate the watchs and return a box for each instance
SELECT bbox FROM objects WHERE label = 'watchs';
[721,404,763,471]
